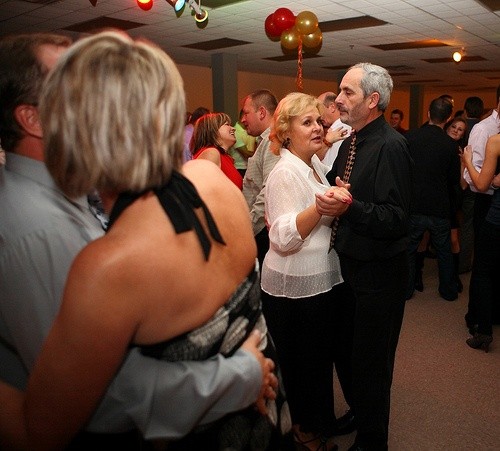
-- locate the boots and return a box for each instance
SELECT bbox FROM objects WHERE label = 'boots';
[451,251,463,293]
[415,249,424,292]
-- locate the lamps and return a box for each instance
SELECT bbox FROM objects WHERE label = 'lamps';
[453,47,464,62]
[138,0,208,22]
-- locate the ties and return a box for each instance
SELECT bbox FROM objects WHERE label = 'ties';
[328,134,356,254]
[88,190,110,230]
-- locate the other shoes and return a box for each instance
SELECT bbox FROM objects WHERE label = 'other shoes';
[465,314,478,335]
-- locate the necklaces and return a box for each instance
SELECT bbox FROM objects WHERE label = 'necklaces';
[308,164,312,167]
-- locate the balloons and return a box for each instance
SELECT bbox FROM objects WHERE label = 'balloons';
[265,7,322,50]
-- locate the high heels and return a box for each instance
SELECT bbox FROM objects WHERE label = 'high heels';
[467,333,492,353]
[294,432,339,451]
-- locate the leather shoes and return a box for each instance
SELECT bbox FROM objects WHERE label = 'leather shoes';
[332,409,358,435]
[347,445,389,451]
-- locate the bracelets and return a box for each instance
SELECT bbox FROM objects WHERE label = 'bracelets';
[323,138,332,147]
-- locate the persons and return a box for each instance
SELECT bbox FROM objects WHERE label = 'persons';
[458,86,500,354]
[315,62,407,451]
[0,28,294,451]
[185,107,211,160]
[406,95,483,301]
[189,112,243,192]
[317,92,354,168]
[227,108,257,179]
[261,92,352,451]
[390,109,410,148]
[241,90,281,276]
[316,126,349,162]
